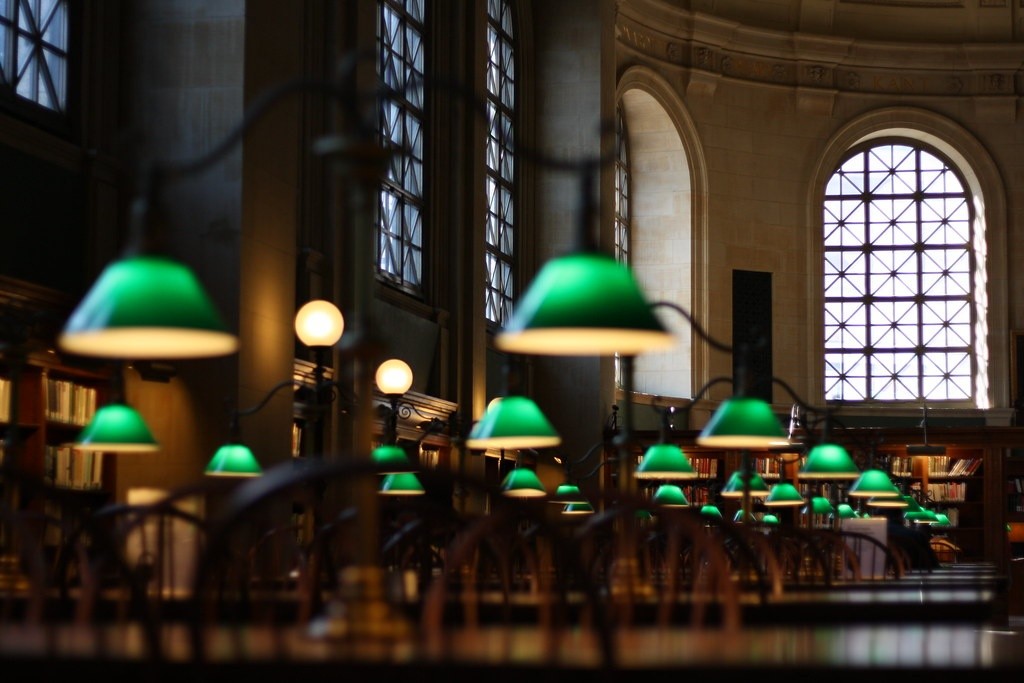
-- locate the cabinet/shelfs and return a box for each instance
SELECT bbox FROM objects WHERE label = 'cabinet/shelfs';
[0,347,106,579]
[600,424,1024,632]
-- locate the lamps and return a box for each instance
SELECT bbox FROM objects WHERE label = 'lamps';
[60,252,955,625]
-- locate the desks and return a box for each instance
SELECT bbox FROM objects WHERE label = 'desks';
[0,565,1024,683]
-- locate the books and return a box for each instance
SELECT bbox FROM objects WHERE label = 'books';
[637,456,718,507]
[881,455,983,529]
[0,378,103,489]
[753,457,851,529]
